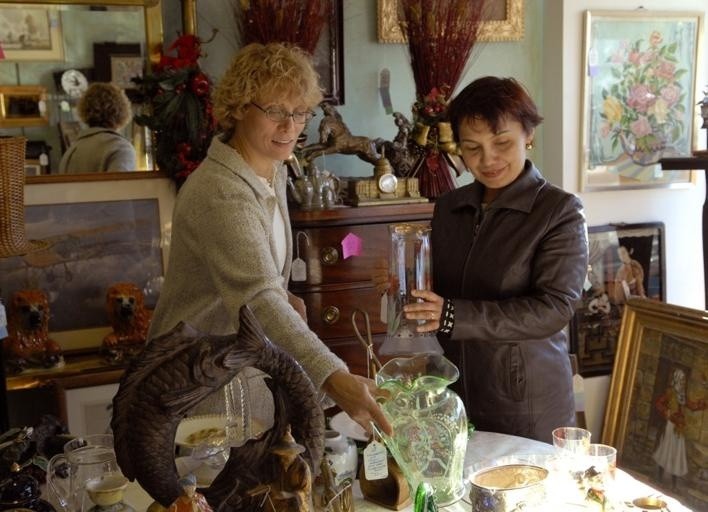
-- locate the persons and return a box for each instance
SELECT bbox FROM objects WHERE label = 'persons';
[611,240,646,316]
[167,470,211,511]
[266,429,312,512]
[144,41,398,442]
[401,77,590,449]
[56,83,141,176]
[650,366,708,495]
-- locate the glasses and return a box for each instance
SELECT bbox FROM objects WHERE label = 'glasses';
[250,102,316,123]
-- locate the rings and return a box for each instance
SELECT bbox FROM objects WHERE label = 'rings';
[429,311,437,321]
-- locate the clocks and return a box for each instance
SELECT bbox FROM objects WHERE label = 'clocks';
[52,66,96,100]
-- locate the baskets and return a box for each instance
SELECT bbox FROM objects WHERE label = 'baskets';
[0,136,29,257]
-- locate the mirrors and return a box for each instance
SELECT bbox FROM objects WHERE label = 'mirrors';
[1,1,168,185]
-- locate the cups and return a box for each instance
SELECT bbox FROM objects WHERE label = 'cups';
[551,425,619,487]
[45,432,125,512]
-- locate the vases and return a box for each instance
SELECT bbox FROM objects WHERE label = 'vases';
[372,349,468,508]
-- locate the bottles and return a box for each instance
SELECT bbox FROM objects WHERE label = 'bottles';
[299,175,314,207]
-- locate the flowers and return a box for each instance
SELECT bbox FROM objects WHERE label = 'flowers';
[596,31,691,166]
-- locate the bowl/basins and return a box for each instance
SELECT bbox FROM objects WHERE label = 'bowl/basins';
[87,502,135,512]
[80,474,127,506]
[467,462,551,512]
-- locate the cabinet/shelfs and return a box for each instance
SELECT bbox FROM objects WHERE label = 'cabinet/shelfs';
[282,200,443,380]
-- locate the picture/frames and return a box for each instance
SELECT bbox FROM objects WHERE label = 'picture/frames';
[1,4,68,65]
[23,159,46,177]
[0,82,50,130]
[309,0,347,108]
[567,221,667,381]
[1,176,180,360]
[54,369,125,439]
[597,293,708,512]
[375,1,526,46]
[577,6,705,194]
[107,53,143,88]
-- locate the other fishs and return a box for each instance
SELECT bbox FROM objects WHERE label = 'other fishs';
[109,303,327,512]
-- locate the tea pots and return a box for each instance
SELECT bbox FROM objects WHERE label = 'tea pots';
[287,164,340,208]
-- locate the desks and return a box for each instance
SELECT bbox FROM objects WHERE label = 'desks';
[4,358,116,394]
[35,423,697,512]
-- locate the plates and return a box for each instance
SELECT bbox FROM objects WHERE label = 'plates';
[328,410,371,443]
[172,412,268,449]
